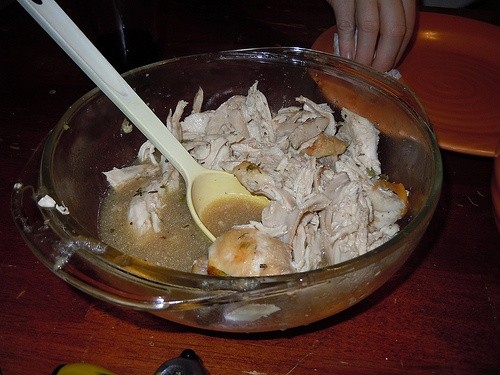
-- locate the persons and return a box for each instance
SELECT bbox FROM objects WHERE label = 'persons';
[321,0,416,76]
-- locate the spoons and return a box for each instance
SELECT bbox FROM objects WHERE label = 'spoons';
[16,1,271,242]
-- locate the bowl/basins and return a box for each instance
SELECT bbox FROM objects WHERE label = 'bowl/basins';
[10,46,441,333]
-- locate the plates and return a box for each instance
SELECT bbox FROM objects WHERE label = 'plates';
[308,11,500,157]
